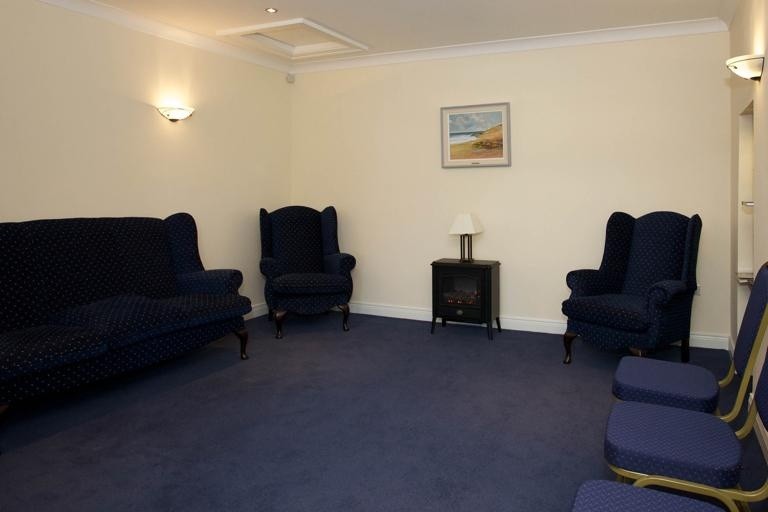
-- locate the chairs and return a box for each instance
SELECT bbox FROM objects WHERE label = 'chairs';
[570,480,727,512]
[259,204,356,339]
[602,346,768,512]
[559,210,702,365]
[608,260,768,424]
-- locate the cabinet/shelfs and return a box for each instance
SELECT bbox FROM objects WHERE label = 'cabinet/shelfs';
[430,257,502,341]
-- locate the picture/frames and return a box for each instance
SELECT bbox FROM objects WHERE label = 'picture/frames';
[440,101,512,168]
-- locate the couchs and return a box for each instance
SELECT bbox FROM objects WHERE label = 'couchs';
[0,212,252,413]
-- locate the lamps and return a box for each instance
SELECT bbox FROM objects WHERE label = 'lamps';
[448,213,484,264]
[725,54,765,82]
[156,106,195,122]
